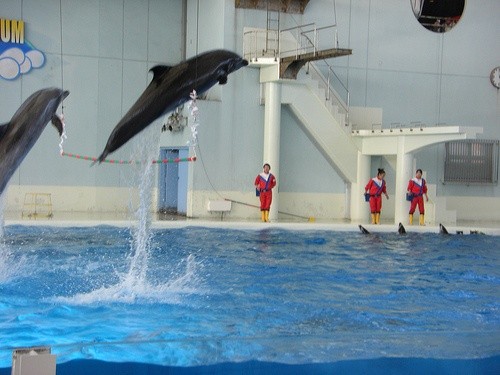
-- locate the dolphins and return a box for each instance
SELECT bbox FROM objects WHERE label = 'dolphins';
[89,49,249,168]
[440,223,448,234]
[359,225,370,234]
[0,87,71,198]
[398,222,406,235]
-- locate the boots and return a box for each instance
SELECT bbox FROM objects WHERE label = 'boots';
[372,214,376,224]
[261,210,265,222]
[265,210,271,222]
[409,214,413,225]
[420,214,426,226]
[376,214,380,224]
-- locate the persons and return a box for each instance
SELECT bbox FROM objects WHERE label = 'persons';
[406,169,429,226]
[364,168,390,225]
[254,163,276,223]
